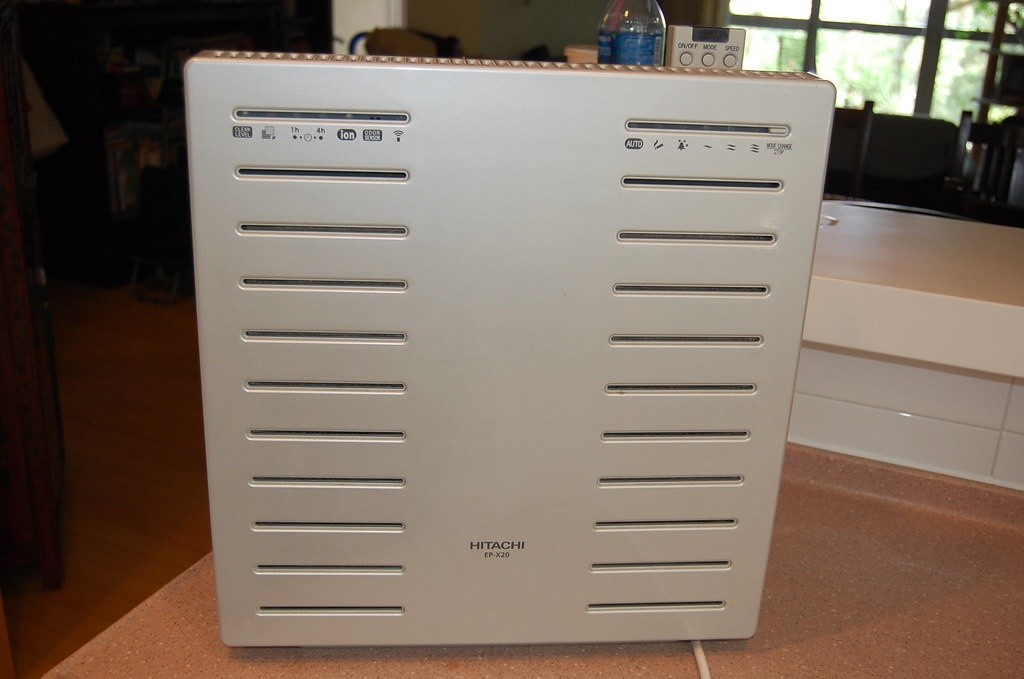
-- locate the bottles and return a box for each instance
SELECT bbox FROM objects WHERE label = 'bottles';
[598,0,666,66]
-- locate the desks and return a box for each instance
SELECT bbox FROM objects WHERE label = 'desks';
[45,441,1024,679]
